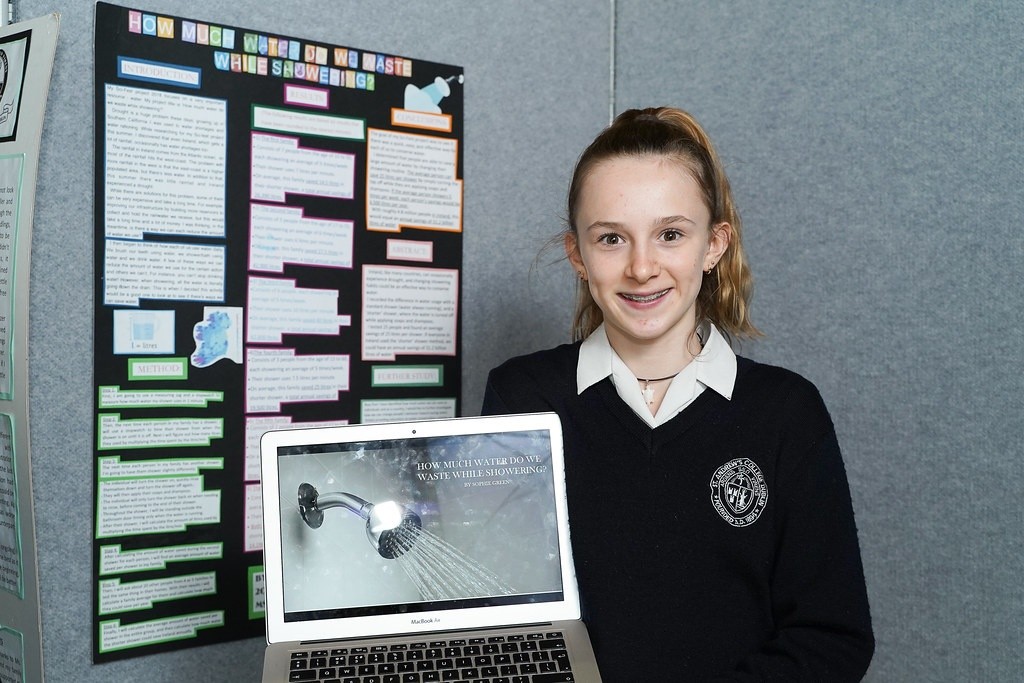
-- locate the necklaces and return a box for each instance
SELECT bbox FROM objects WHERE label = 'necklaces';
[636,333,704,406]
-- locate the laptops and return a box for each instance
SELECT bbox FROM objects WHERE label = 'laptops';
[260,410,603,683]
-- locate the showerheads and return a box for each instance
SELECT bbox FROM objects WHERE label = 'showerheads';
[297,481,425,559]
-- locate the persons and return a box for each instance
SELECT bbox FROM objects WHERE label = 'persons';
[482,108,875,683]
